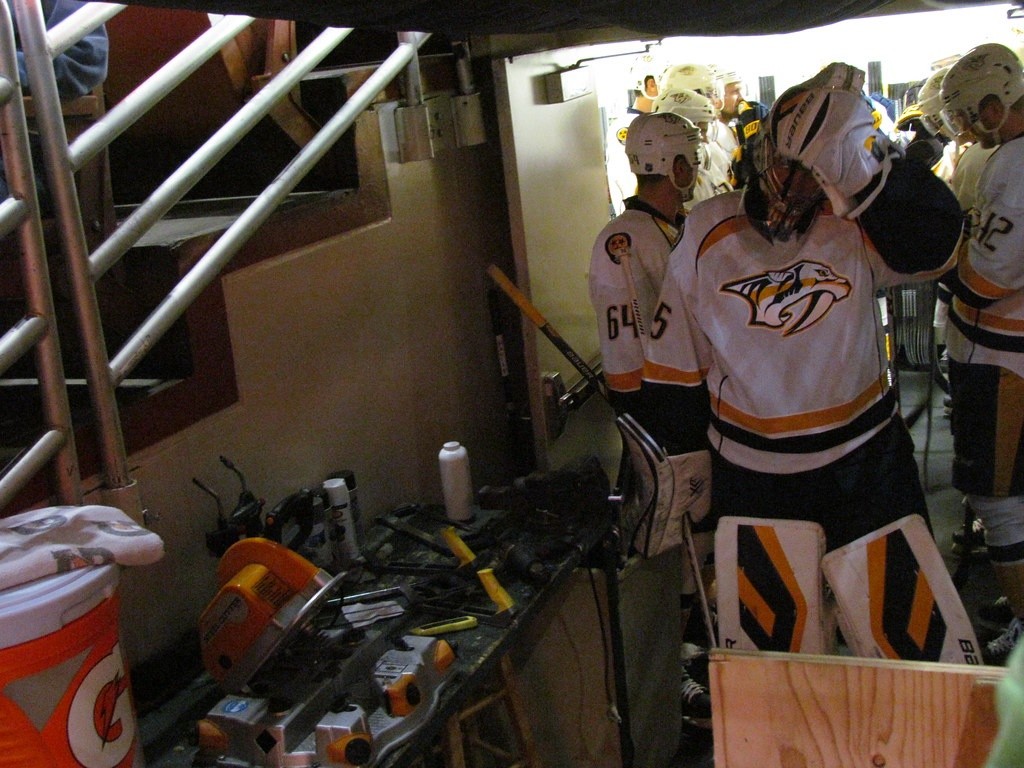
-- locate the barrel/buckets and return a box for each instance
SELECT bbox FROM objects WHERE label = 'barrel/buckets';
[0,564,146,768]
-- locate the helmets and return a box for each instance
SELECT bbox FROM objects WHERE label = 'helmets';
[709,68,741,111]
[632,44,678,100]
[652,88,714,127]
[625,113,703,201]
[893,42,1024,144]
[768,62,876,183]
[667,65,712,94]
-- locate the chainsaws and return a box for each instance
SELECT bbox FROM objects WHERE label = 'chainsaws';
[197,531,348,698]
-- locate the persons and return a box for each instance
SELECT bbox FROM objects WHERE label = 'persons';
[7,0,109,99]
[933,43,1024,667]
[634,62,964,728]
[606,62,1001,553]
[589,112,713,725]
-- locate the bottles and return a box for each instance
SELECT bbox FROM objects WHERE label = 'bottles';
[303,470,364,570]
[439,442,475,521]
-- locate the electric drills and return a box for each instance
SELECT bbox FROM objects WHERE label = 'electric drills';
[493,530,575,584]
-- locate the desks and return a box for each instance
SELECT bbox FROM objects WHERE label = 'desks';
[138,501,634,768]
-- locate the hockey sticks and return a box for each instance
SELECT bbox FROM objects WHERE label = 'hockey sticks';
[488,281,515,424]
[930,280,950,393]
[479,255,675,560]
[887,287,901,416]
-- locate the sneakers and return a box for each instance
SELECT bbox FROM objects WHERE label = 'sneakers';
[981,596,1024,660]
[681,642,712,729]
